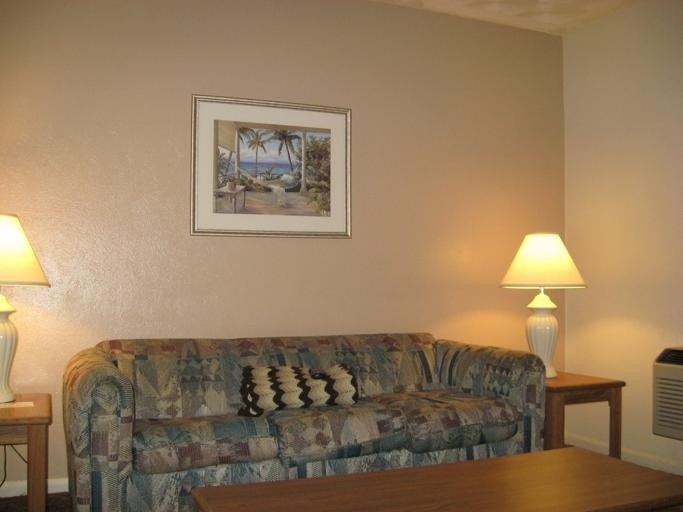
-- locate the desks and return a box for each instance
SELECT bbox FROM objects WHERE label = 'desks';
[192,446,683,511]
[0,393,52,512]
[545,371,626,458]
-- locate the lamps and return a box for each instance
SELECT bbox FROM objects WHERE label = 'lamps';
[62,332,546,512]
[0,213,50,404]
[500,233,587,378]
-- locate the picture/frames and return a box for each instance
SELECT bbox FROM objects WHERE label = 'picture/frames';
[190,94,352,239]
